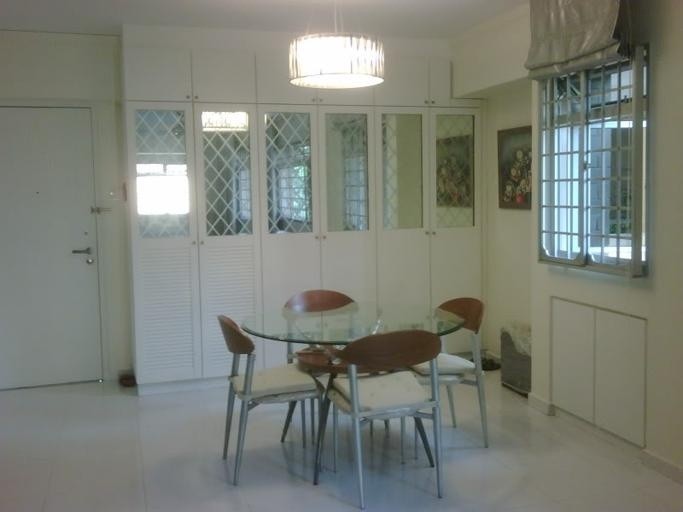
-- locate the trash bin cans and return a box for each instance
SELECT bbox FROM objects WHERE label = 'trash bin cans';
[501,320,531,395]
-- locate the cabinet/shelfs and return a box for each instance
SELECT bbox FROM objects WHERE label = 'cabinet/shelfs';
[258,33,376,370]
[377,40,485,352]
[119,26,257,396]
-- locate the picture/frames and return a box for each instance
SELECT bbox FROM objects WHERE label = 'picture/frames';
[436,136,472,208]
[497,126,532,210]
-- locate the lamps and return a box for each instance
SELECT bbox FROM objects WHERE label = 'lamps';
[202,112,249,133]
[288,8,387,89]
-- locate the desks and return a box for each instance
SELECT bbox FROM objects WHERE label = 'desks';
[242,305,466,485]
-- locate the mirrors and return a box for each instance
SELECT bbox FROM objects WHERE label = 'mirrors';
[381,113,423,230]
[325,112,369,233]
[264,111,313,234]
[135,109,190,239]
[434,114,474,228]
[202,110,253,237]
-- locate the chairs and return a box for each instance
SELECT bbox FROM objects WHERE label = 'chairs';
[279,290,375,447]
[328,328,445,510]
[408,297,489,457]
[217,315,339,485]
